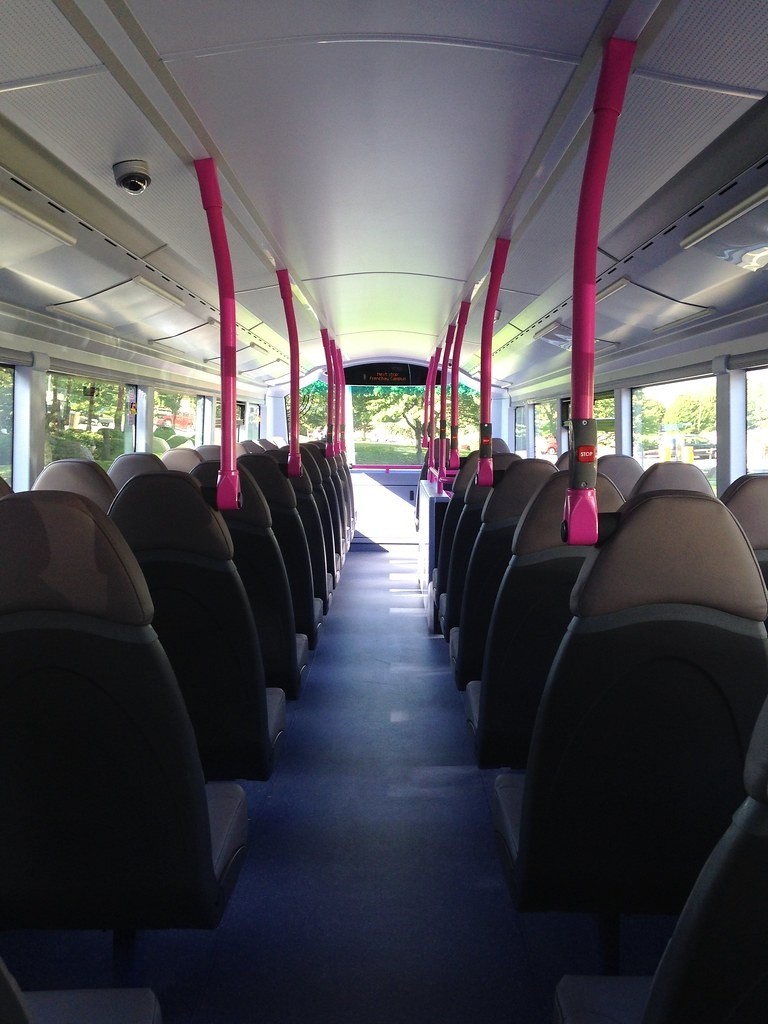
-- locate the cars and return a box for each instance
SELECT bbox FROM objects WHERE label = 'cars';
[153,404,194,429]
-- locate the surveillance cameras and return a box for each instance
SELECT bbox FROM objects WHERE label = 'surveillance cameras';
[113,160,152,196]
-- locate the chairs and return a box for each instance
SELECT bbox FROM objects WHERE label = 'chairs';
[0,437,356,1024]
[416,438,768,1024]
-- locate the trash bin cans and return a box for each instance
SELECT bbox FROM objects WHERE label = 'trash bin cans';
[659,446,671,462]
[69,412,81,429]
[683,446,694,463]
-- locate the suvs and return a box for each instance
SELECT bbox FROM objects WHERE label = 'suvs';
[671,433,717,459]
